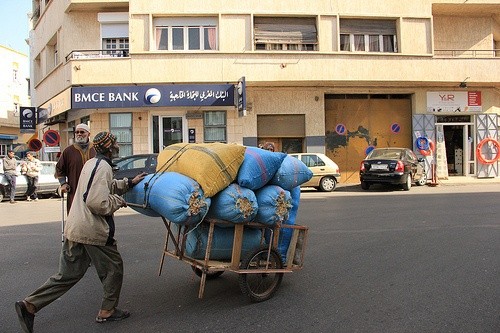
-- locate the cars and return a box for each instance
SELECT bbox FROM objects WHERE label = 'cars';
[0,161,69,202]
[359,147,427,191]
[286,153,341,192]
[111,152,159,180]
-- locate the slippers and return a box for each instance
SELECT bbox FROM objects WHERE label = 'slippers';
[15,301,35,333]
[96,308,129,322]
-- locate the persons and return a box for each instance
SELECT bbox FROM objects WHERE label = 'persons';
[20,151,43,201]
[263,142,275,152]
[55,123,97,266]
[3,150,18,203]
[15,131,148,333]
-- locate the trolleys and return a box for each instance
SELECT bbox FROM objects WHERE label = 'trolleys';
[123,202,310,303]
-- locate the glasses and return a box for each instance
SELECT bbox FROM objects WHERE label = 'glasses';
[75,131,88,134]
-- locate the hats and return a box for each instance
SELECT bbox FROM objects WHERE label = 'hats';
[93,132,117,153]
[76,123,90,133]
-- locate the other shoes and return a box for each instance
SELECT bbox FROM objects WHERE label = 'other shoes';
[35,198,38,200]
[27,195,32,201]
[10,200,15,203]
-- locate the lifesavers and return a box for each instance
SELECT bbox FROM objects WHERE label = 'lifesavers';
[417,137,434,155]
[477,138,500,164]
